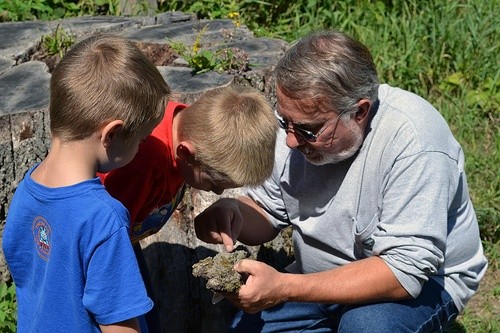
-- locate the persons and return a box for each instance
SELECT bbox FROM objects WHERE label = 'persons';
[193,31,489,333]
[2,34,174,333]
[95,85,277,333]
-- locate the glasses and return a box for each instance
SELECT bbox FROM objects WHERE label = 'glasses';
[272,102,355,143]
